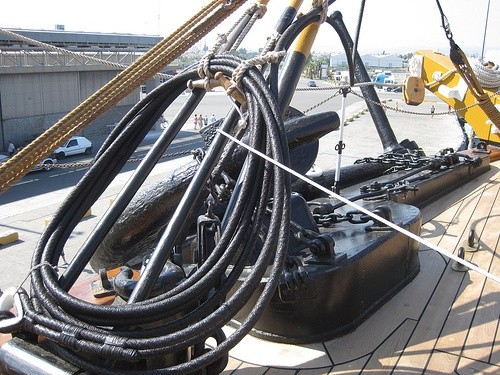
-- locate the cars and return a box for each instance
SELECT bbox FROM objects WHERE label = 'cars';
[53,135,93,160]
[308,81,316,87]
[28,157,57,173]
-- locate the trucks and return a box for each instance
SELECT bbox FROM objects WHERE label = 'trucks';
[375,71,405,93]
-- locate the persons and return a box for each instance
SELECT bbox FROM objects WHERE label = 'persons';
[194,114,216,130]
[431,105,436,118]
[8,140,16,158]
